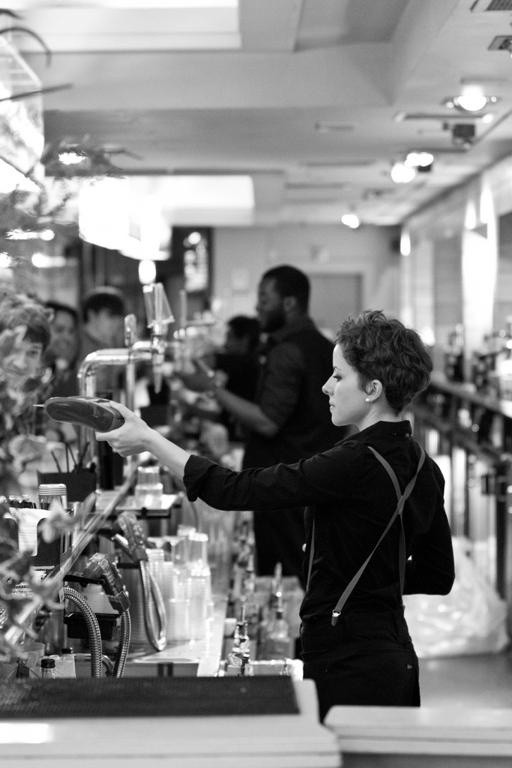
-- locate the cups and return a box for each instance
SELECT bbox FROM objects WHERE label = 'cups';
[141,282,176,328]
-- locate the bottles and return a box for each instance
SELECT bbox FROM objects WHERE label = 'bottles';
[164,524,212,644]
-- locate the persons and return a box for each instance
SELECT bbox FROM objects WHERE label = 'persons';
[40,300,84,404]
[74,286,125,369]
[212,262,350,593]
[93,308,454,725]
[1,317,47,457]
[212,316,263,441]
